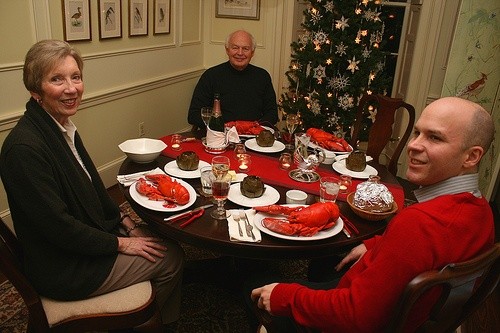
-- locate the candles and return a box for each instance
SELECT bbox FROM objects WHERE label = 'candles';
[169,135,183,151]
[237,155,252,172]
[338,174,352,194]
[279,153,291,169]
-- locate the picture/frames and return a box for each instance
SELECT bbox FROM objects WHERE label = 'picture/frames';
[215,0,260,21]
[128,0,148,37]
[153,0,171,35]
[98,0,122,40]
[62,0,92,42]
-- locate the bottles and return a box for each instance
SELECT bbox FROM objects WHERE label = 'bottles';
[234,143,246,160]
[209,94,226,132]
[170,135,183,151]
[339,174,352,195]
[368,175,381,185]
[277,152,292,170]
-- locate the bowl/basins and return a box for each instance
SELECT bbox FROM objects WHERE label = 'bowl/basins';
[286,189,308,207]
[118,137,169,164]
[319,151,336,165]
[346,190,398,222]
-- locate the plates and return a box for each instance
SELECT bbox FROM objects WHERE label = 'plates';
[227,183,280,207]
[129,176,197,213]
[255,204,344,241]
[227,122,378,179]
[163,160,211,179]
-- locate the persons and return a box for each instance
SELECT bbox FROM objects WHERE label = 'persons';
[187,30,278,129]
[251,96,500,333]
[0,40,187,333]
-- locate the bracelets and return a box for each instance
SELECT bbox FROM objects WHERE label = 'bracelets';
[126,223,142,234]
[120,214,130,227]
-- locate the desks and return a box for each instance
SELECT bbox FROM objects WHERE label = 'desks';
[118,128,406,333]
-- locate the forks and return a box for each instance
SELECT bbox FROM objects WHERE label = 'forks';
[234,208,252,237]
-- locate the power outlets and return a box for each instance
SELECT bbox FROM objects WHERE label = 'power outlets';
[139,122,145,137]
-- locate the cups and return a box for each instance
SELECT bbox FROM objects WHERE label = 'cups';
[200,166,212,195]
[319,176,341,205]
[200,107,213,125]
[237,154,252,173]
[295,132,310,164]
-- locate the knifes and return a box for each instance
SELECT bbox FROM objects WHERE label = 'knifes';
[163,204,213,222]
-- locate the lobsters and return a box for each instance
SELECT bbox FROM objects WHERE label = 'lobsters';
[253,201,359,237]
[224,120,266,135]
[136,174,190,205]
[306,128,352,152]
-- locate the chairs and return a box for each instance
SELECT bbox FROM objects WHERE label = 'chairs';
[371,244,500,333]
[0,218,156,333]
[352,93,416,176]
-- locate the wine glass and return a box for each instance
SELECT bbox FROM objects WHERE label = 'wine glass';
[210,156,232,220]
